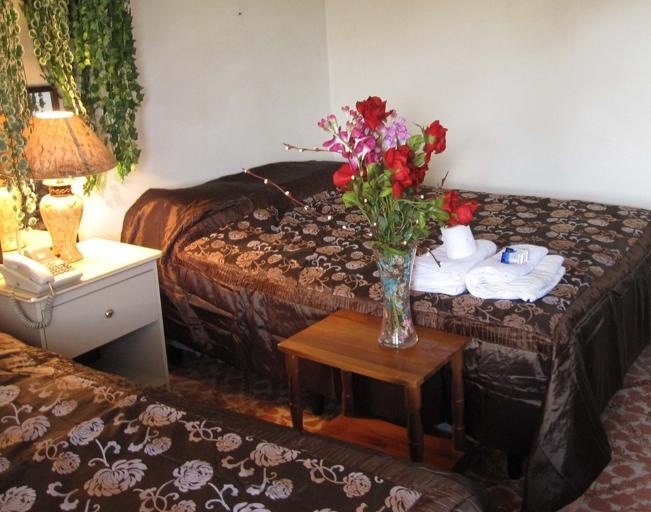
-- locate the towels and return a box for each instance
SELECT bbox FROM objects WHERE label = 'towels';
[405,236,498,297]
[482,236,552,280]
[466,245,569,307]
[439,225,477,259]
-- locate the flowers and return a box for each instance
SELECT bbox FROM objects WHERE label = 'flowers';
[239,94,482,339]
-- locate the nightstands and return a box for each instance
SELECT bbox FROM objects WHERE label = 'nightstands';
[1,237,175,392]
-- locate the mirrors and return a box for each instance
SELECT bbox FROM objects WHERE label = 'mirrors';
[0,85,83,269]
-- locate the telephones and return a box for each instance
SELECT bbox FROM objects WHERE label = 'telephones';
[0,245,83,296]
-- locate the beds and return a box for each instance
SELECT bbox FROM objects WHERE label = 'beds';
[0,328,491,511]
[116,156,651,481]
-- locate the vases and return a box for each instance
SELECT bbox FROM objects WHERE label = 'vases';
[367,239,426,350]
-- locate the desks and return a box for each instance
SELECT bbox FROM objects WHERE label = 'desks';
[273,305,474,476]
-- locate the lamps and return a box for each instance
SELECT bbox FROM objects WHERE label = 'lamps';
[0,114,29,254]
[0,106,121,266]
